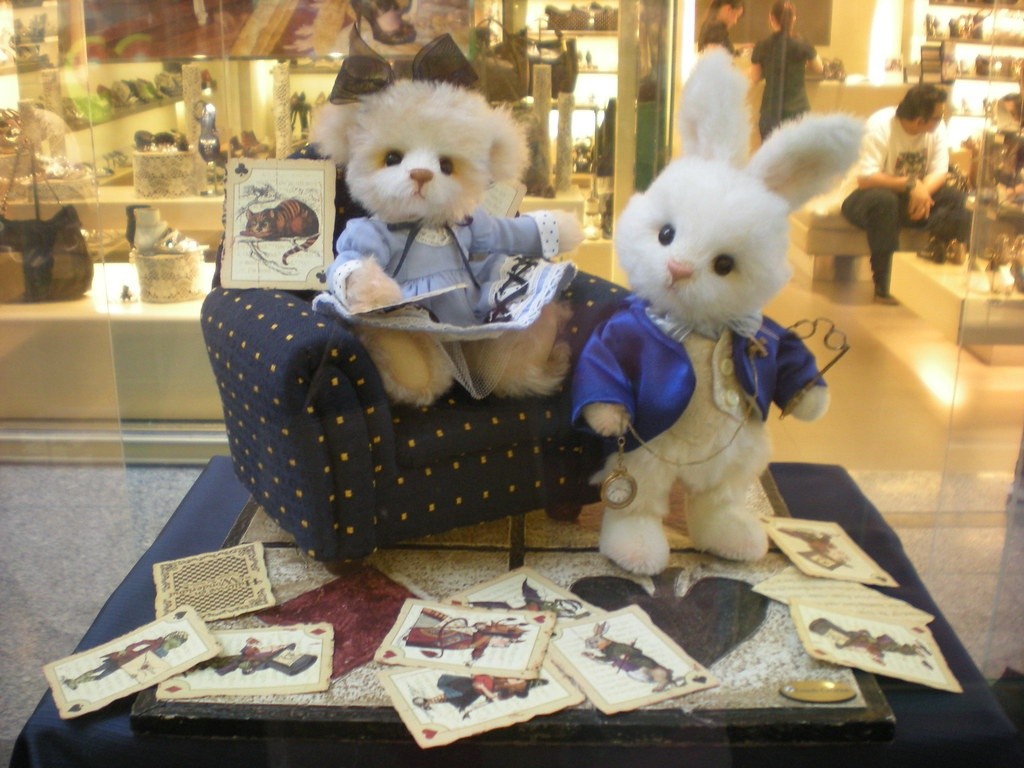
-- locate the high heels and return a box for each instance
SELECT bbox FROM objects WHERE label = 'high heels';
[198,104,220,162]
[349,0,417,45]
[103,151,132,168]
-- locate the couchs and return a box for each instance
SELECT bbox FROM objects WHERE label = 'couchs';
[200,144,638,562]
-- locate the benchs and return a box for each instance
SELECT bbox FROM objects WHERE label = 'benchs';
[790,195,1023,281]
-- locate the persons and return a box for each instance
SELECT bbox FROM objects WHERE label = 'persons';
[350,0,417,44]
[699,0,826,144]
[838,83,968,304]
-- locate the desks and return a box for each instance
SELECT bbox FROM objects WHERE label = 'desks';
[9,456,1023,768]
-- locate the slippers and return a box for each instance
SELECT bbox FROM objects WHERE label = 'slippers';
[132,228,209,254]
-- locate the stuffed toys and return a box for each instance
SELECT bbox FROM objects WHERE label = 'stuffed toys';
[571,44,864,575]
[310,78,584,407]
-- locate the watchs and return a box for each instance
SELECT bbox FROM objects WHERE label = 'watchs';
[904,176,917,194]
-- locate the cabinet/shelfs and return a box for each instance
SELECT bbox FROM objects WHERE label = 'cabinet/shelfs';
[0,0,621,190]
[901,0,1024,119]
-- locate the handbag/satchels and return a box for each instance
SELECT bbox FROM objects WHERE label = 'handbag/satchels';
[545,5,590,30]
[516,18,578,98]
[592,2,618,30]
[0,203,94,303]
[948,15,983,38]
[976,54,1013,76]
[476,19,518,97]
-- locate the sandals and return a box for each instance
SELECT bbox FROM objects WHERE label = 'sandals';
[946,238,967,265]
[917,236,946,264]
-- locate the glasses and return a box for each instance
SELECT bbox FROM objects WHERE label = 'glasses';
[930,116,941,122]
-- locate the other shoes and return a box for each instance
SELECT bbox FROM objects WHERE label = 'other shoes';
[875,289,895,304]
[230,130,268,157]
[81,162,113,176]
[97,74,182,107]
